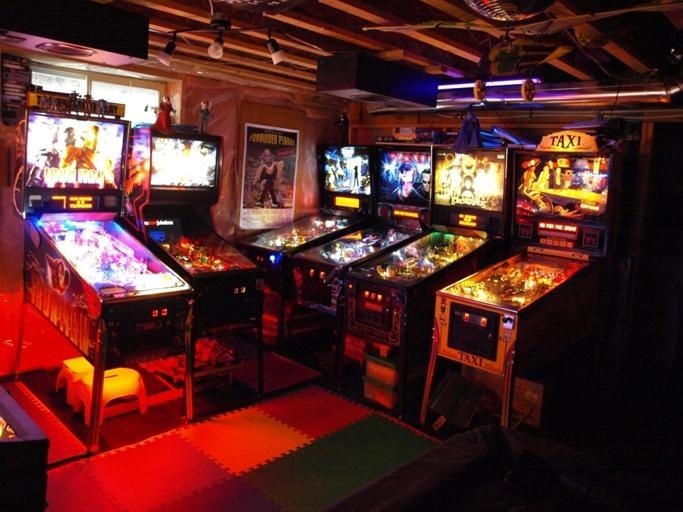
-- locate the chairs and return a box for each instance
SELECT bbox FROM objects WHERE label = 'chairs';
[319,423,681,512]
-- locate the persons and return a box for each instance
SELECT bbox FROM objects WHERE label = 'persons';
[557,167,574,188]
[515,156,585,220]
[461,175,477,197]
[68,90,106,118]
[407,167,430,208]
[252,148,284,208]
[198,99,216,134]
[150,95,177,136]
[25,124,116,189]
[390,159,429,208]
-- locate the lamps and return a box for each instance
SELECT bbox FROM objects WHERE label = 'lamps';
[153,11,287,68]
